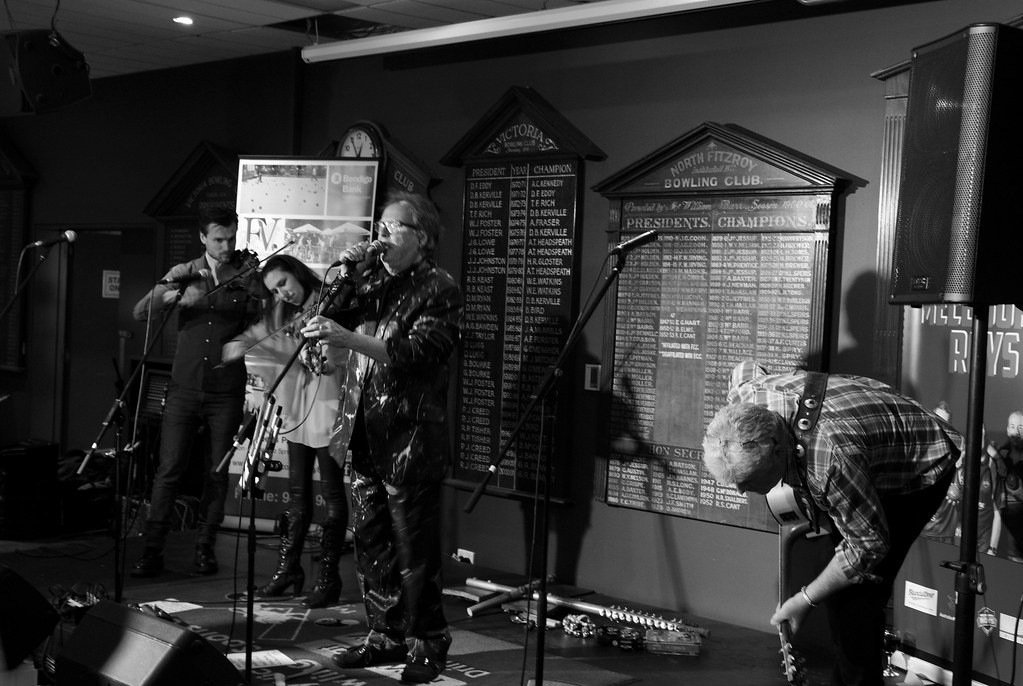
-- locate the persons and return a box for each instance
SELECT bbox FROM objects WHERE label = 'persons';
[133,191,459,683]
[701,358,961,686]
[920,400,1023,564]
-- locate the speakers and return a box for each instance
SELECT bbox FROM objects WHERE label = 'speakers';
[37,592,248,686]
[0,562,59,669]
[888,22,1023,311]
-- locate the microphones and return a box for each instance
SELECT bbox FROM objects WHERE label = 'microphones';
[157,269,213,284]
[611,230,658,255]
[28,230,79,249]
[332,241,387,268]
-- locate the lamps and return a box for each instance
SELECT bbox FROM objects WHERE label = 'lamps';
[300,1,742,66]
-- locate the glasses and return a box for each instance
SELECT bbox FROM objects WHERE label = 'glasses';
[373,220,419,234]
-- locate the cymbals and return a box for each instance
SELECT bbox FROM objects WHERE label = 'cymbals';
[593,625,644,650]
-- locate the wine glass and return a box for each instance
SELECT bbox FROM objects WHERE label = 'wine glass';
[882,626,901,677]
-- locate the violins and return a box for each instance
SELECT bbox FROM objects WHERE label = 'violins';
[292,288,327,376]
[217,249,274,301]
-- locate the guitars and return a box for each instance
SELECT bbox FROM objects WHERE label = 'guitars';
[765,481,822,682]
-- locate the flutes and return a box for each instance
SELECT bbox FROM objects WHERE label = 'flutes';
[466,576,712,638]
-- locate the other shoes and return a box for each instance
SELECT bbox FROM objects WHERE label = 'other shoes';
[400,632,452,684]
[183,543,220,577]
[130,547,165,577]
[332,624,409,669]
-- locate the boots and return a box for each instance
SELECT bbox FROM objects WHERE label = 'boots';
[299,514,349,608]
[255,512,311,597]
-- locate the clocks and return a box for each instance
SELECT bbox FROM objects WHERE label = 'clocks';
[335,121,384,162]
